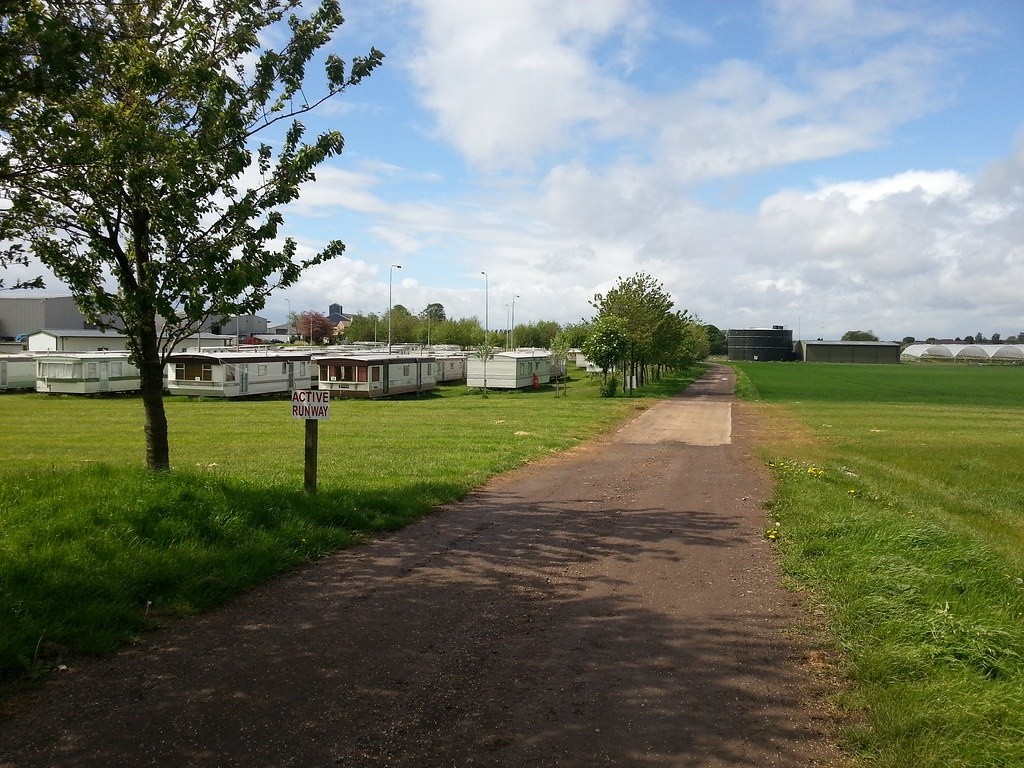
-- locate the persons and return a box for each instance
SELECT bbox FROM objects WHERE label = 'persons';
[532,373,539,390]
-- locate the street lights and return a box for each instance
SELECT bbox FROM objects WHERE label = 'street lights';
[285,299,291,343]
[389,265,401,356]
[505,304,510,352]
[480,271,488,351]
[511,296,520,349]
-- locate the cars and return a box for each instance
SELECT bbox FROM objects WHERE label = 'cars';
[270,339,284,344]
